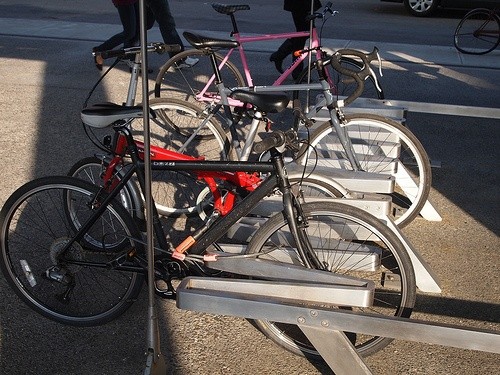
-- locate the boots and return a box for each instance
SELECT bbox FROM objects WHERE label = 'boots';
[269,38,294,74]
[292,56,312,82]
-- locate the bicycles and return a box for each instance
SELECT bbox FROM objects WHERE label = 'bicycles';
[1,0,433,363]
[453,7,500,55]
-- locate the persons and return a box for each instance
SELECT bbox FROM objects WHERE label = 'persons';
[93,0,200,74]
[270,0,322,82]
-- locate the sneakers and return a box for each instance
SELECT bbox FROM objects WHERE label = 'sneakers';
[175,56,200,70]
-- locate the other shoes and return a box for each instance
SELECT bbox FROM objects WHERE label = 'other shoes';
[130,65,153,74]
[93,47,104,71]
